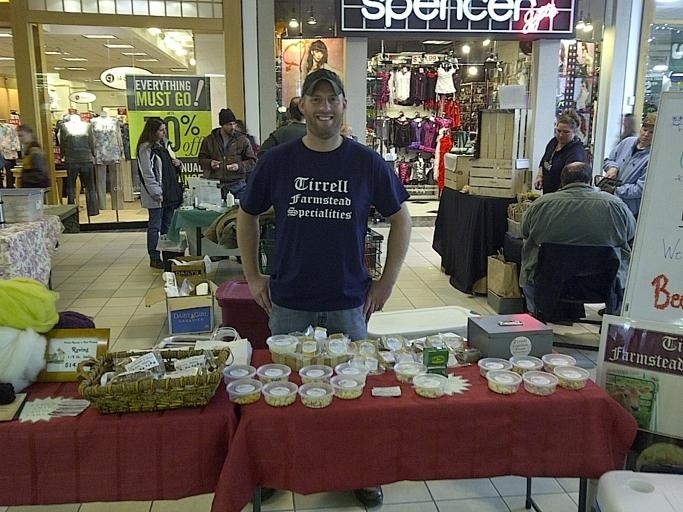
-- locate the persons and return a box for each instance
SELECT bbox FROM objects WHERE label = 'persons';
[579,43,594,73]
[255,97,315,153]
[14,126,59,251]
[558,44,565,72]
[600,111,655,218]
[520,162,639,312]
[136,117,185,269]
[302,41,338,80]
[618,114,640,142]
[238,71,412,506]
[575,79,588,107]
[199,108,257,204]
[534,110,589,197]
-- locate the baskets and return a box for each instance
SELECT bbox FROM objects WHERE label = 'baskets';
[76,347,232,414]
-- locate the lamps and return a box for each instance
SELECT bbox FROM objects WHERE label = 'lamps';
[575,3,585,30]
[581,1,594,33]
[306,6,317,25]
[289,2,298,28]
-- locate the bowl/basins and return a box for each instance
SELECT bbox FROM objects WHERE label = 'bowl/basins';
[541,353,576,372]
[522,371,557,395]
[297,382,335,409]
[265,334,298,355]
[256,363,290,383]
[298,326,354,360]
[393,361,427,384]
[225,379,262,404]
[261,381,298,407]
[334,362,370,379]
[222,364,256,383]
[329,375,365,399]
[554,366,588,389]
[355,338,386,377]
[386,331,469,368]
[477,357,511,376]
[412,373,449,399]
[509,355,543,374]
[485,371,521,394]
[297,364,333,383]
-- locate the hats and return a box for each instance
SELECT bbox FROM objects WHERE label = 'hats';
[302,69,347,98]
[219,108,236,125]
[642,110,657,124]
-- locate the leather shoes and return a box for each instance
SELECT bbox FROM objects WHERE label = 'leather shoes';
[355,484,384,505]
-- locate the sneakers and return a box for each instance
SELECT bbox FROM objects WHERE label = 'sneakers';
[149,256,163,269]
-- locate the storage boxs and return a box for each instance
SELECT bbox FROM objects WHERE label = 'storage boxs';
[171,253,212,296]
[467,313,554,363]
[145,279,218,337]
[2,187,44,224]
[487,289,527,315]
[214,278,272,348]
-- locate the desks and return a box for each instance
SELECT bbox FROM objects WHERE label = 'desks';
[0,345,241,498]
[243,346,604,512]
[0,214,63,289]
[176,206,275,256]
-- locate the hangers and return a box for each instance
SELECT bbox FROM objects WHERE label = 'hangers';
[375,108,453,154]
[389,155,439,191]
[371,52,461,104]
